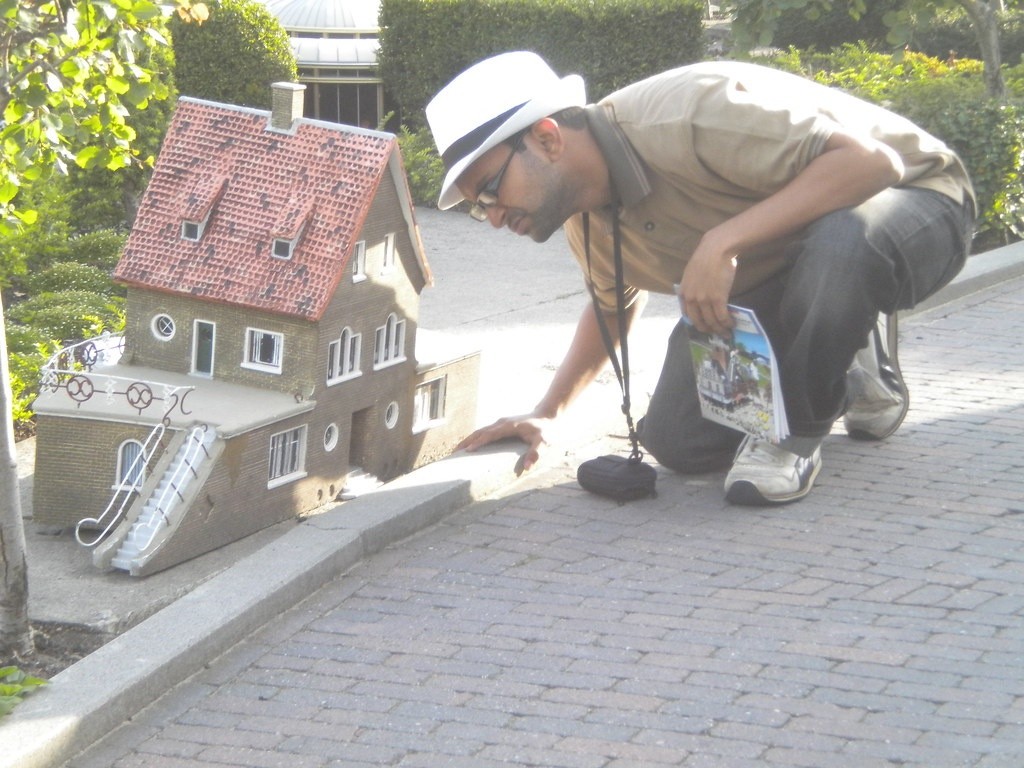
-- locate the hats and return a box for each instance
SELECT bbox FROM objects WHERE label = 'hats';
[423,48,587,214]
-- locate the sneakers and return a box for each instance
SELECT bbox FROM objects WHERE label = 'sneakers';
[841,305,912,444]
[722,420,824,509]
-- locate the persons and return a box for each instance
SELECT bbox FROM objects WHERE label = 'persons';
[423,49,976,508]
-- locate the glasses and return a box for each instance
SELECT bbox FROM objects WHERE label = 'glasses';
[467,127,530,223]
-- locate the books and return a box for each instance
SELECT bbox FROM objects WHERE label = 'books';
[672,281,791,446]
